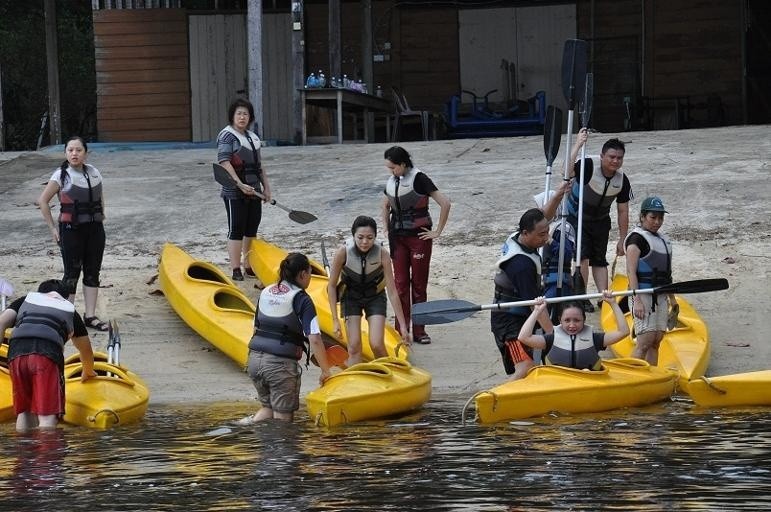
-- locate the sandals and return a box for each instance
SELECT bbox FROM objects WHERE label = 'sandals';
[413,334,431,344]
[83,313,108,331]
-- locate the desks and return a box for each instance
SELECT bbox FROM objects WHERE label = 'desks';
[296,86,396,145]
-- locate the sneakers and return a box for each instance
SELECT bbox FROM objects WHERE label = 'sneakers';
[232,267,244,281]
[246,268,255,276]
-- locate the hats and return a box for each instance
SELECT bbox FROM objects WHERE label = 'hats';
[641,196,668,213]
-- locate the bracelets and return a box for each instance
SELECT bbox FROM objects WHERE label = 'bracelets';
[332,317,338,320]
[47,226,53,231]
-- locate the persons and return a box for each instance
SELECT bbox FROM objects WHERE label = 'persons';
[622,195,678,366]
[490,177,574,382]
[327,215,413,368]
[517,290,630,371]
[0,279,98,430]
[565,127,634,312]
[215,99,272,281]
[38,136,109,331]
[382,145,451,344]
[238,252,331,425]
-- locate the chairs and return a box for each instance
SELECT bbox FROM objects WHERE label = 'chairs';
[391,87,425,142]
[402,93,429,141]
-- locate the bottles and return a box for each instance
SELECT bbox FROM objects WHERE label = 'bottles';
[306,70,382,97]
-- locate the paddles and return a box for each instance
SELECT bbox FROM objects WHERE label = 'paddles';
[1,280,12,311]
[107,318,115,376]
[574,72,593,308]
[550,40,588,328]
[410,277,729,325]
[320,239,335,284]
[537,107,562,287]
[112,319,121,376]
[211,164,316,226]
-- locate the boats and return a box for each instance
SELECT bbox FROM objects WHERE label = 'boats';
[474,356,676,424]
[248,226,411,358]
[54,346,149,433]
[301,355,437,430]
[600,271,711,384]
[678,368,770,409]
[159,240,268,370]
[0,325,37,421]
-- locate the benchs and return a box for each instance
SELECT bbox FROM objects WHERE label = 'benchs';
[445,89,546,137]
